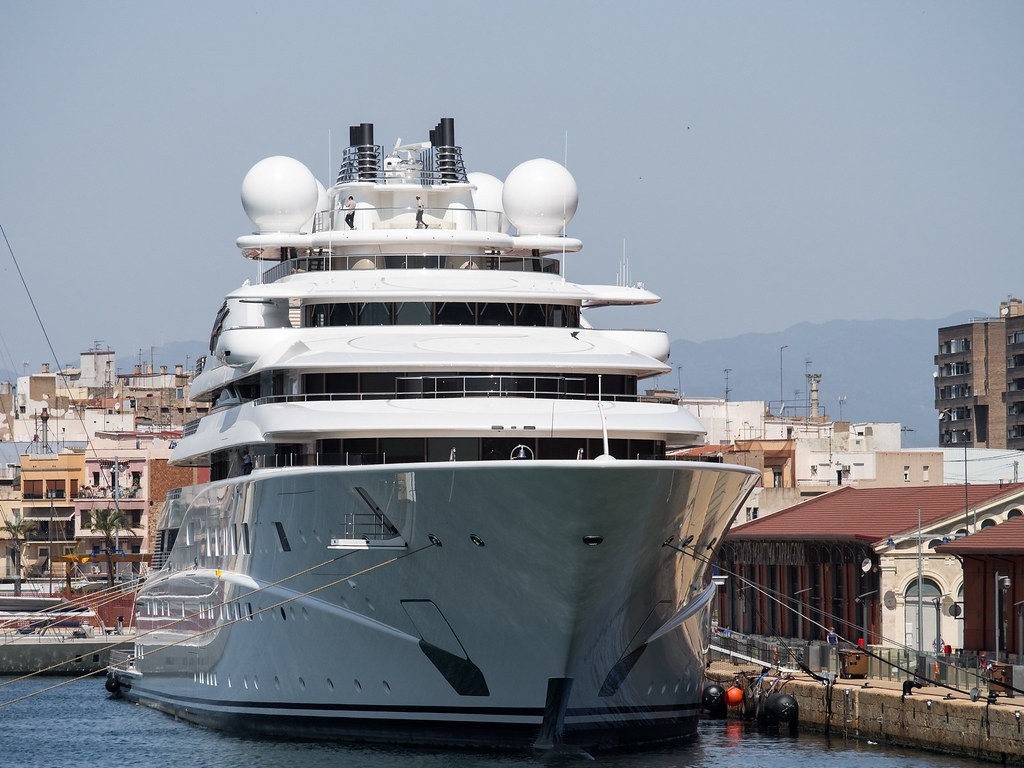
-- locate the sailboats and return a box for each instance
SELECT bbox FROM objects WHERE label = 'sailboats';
[1,223,153,628]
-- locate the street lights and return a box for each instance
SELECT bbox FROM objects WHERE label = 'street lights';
[109,465,126,581]
[678,366,683,398]
[780,345,789,406]
[994,574,1012,661]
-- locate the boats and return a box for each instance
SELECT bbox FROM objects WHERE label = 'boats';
[98,116,762,758]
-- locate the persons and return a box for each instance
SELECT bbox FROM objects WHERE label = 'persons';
[416,194,428,229]
[932,634,945,653]
[723,625,730,637]
[826,627,838,654]
[345,196,357,230]
